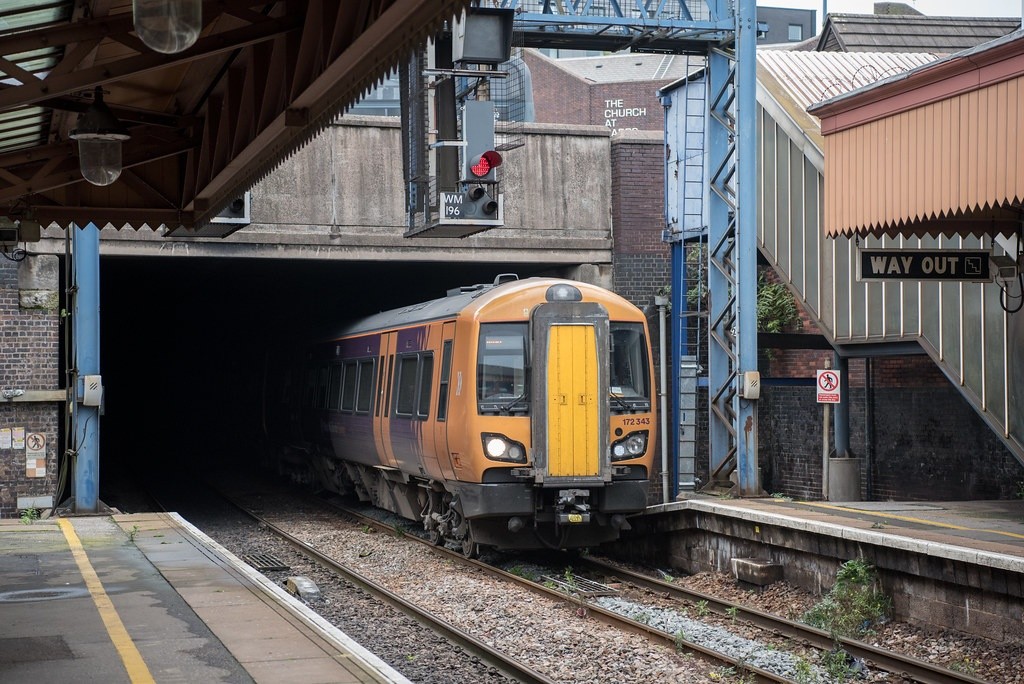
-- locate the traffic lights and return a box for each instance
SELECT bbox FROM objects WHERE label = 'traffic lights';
[470,153,491,176]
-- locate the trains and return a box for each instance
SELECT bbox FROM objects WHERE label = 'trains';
[241,272,662,566]
